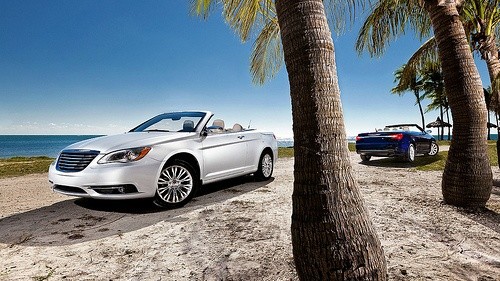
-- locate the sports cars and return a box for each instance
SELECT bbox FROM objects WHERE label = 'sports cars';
[48,110,279,209]
[355,123,440,163]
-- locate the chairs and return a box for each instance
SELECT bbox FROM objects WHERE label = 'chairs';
[378,126,410,131]
[183,120,242,132]
